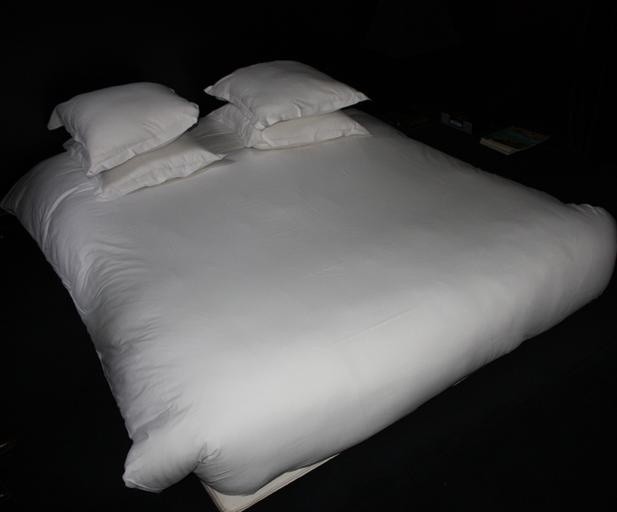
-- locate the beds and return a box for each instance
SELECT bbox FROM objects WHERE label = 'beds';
[0,108,616,512]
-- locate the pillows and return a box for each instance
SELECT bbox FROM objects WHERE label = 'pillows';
[208,104,371,151]
[204,60,370,131]
[64,136,226,202]
[48,83,199,177]
